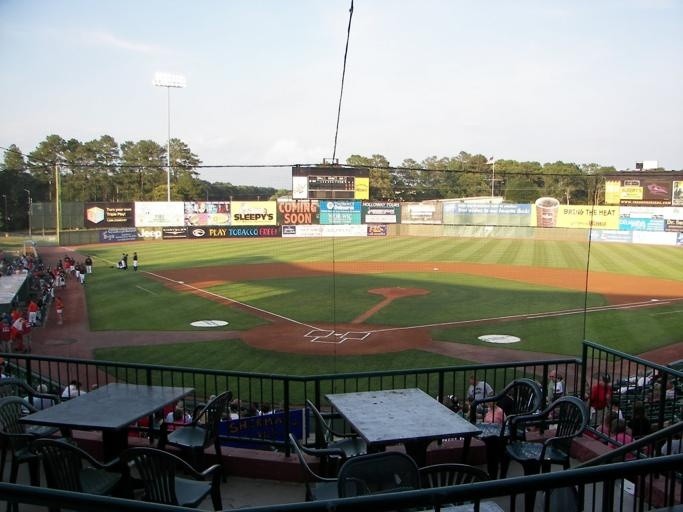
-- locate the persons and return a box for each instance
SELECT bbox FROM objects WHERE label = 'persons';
[549,368,681,461]
[153,394,276,430]
[1,362,87,411]
[438,376,507,425]
[1,252,92,355]
[117,252,138,271]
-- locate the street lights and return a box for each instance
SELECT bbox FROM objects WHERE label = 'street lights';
[23,189,32,238]
[154,83,182,201]
[1,194,8,223]
